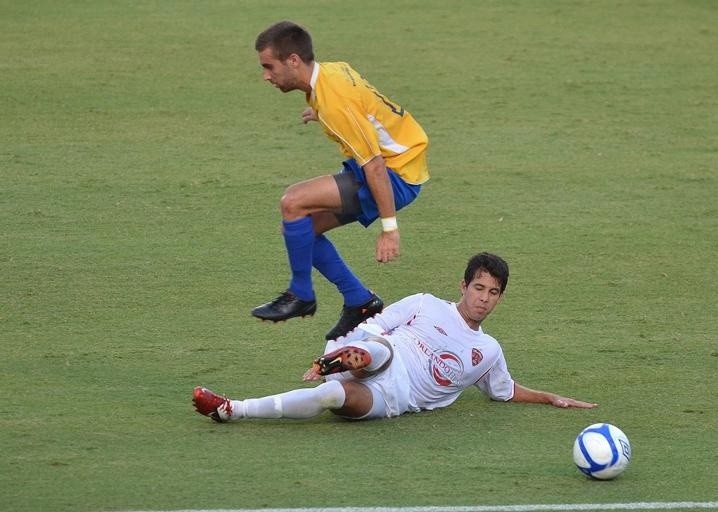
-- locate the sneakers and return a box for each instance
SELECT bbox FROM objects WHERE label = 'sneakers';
[192,385,233,424]
[252,288,318,322]
[326,289,385,341]
[312,346,372,376]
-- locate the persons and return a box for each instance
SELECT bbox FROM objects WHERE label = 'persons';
[190,251,600,424]
[248,19,430,342]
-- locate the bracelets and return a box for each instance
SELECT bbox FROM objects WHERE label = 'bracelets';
[379,215,398,234]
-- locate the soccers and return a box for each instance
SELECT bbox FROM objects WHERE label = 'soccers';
[573,423,631,478]
